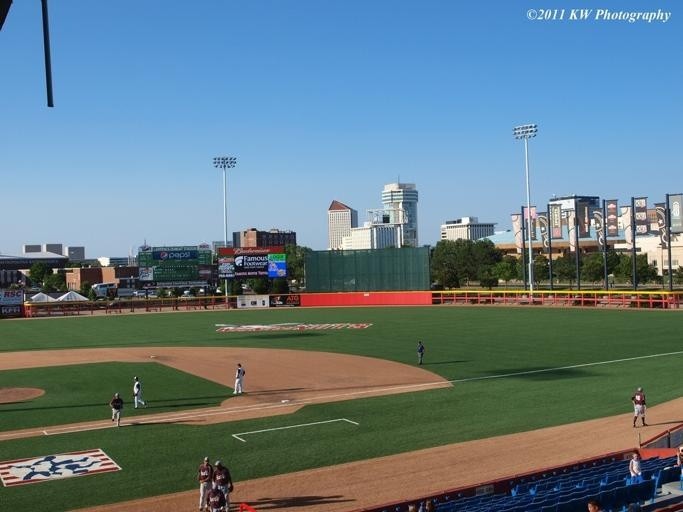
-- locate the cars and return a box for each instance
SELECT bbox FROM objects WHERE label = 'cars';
[133,289,162,300]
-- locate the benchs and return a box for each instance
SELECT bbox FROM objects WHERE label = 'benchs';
[435,457,683,512]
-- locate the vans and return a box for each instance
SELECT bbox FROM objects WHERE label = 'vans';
[91,283,116,298]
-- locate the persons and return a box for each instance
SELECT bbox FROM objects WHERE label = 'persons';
[270,295,283,305]
[132,376,147,409]
[197,457,213,511]
[211,460,233,511]
[672,447,682,468]
[630,386,647,428]
[108,392,122,428]
[407,503,417,512]
[204,481,225,512]
[271,298,280,305]
[587,498,602,512]
[232,363,245,394]
[629,450,642,483]
[424,500,435,512]
[415,340,425,365]
[269,260,278,271]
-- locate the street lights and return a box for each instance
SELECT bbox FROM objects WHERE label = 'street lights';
[213,156,237,309]
[512,124,538,304]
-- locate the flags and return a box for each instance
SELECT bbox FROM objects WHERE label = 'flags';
[0,447,122,487]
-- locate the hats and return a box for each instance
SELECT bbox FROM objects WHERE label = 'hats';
[211,482,218,489]
[215,461,220,466]
[205,457,208,460]
